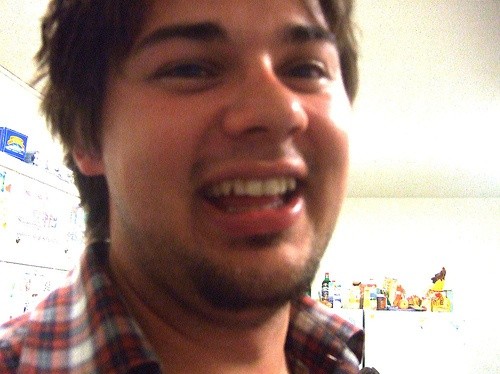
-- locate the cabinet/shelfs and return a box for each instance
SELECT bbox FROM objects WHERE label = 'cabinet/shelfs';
[1,151,86,328]
[327,307,500,374]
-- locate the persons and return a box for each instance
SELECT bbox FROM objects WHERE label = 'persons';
[0,0,380,374]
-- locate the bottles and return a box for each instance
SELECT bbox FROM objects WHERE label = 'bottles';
[322,273,343,310]
[363,285,370,309]
